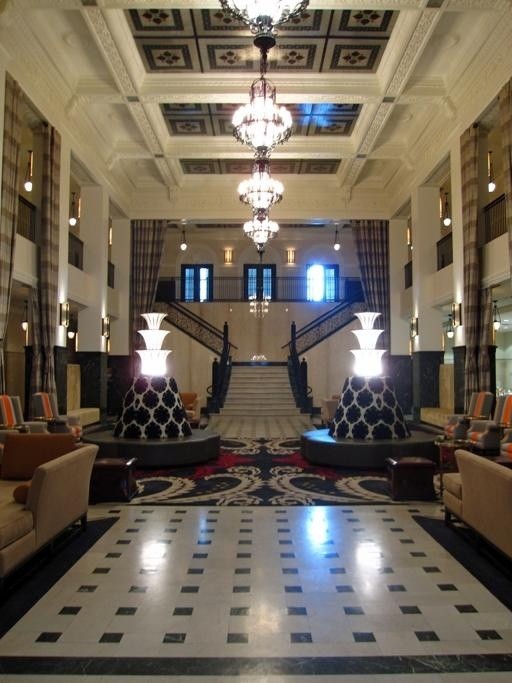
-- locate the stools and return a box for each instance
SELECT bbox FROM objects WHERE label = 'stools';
[89,456,140,506]
[384,455,437,501]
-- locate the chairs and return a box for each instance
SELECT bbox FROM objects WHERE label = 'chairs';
[443,390,495,440]
[463,394,512,452]
[29,392,83,443]
[0,394,49,434]
[499,427,512,457]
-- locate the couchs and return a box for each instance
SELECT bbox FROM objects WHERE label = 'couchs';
[432,449,512,560]
[1,432,98,579]
[179,392,201,421]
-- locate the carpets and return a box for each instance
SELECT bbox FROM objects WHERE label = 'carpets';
[113,436,441,505]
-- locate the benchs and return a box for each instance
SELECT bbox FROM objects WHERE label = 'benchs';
[82,428,219,465]
[300,428,441,468]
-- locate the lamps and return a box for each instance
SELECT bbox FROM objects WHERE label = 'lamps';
[133,310,173,375]
[21,299,29,331]
[443,191,451,226]
[69,191,78,225]
[237,161,284,208]
[241,212,282,256]
[230,58,295,158]
[410,316,419,338]
[23,150,33,192]
[286,248,297,268]
[59,301,70,328]
[452,302,463,327]
[222,244,235,267]
[334,224,341,251]
[492,300,502,331]
[217,0,311,51]
[102,317,110,337]
[349,310,391,378]
[446,314,454,339]
[180,224,188,251]
